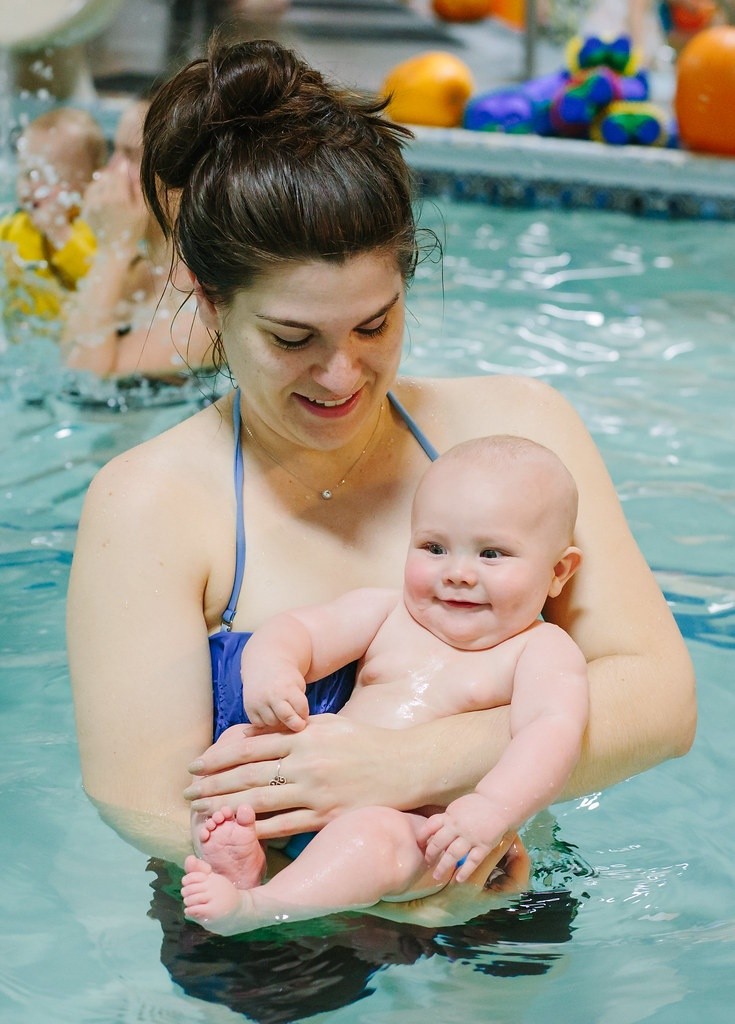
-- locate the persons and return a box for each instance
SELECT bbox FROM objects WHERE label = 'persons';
[179,434,591,938]
[70,41,699,861]
[0,111,156,322]
[64,97,286,380]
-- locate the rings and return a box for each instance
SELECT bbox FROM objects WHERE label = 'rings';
[269,757,285,786]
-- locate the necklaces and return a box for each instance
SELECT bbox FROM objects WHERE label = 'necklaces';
[242,404,383,500]
